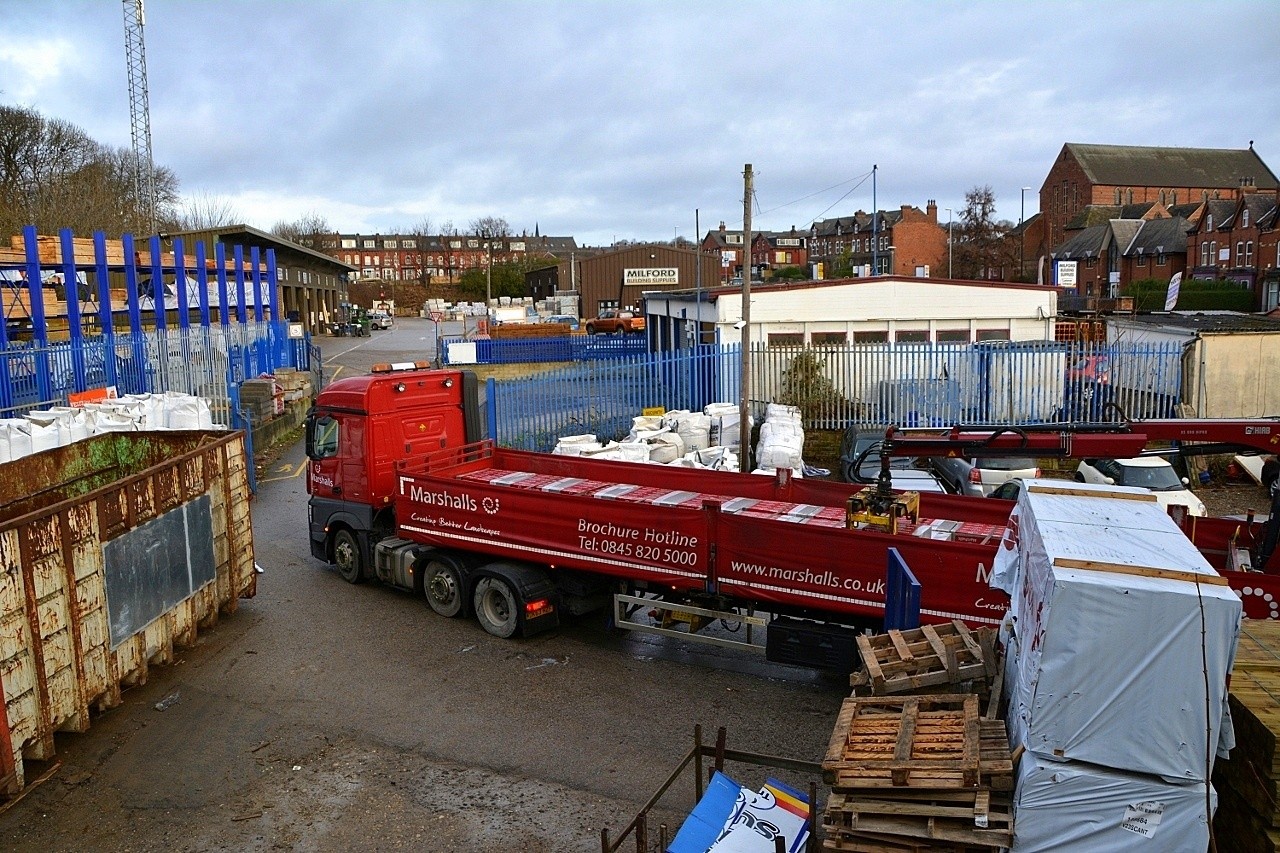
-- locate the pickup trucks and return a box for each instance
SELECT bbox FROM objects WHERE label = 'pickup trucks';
[586,308,645,337]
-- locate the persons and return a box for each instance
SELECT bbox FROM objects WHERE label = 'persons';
[1260,457,1280,491]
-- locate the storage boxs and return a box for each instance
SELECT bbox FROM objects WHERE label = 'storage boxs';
[420,290,587,339]
[201,367,311,430]
[458,467,1005,545]
[711,413,752,446]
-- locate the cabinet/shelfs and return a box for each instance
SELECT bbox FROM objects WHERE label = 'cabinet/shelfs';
[0,225,278,382]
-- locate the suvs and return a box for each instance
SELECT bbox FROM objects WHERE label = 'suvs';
[1076,448,1208,518]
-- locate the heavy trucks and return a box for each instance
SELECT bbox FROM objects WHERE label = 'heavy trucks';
[298,362,1280,674]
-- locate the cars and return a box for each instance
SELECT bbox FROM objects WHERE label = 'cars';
[871,471,948,495]
[838,424,919,485]
[366,314,392,330]
[929,430,1041,499]
[543,314,580,331]
[524,305,542,324]
[1066,355,1109,384]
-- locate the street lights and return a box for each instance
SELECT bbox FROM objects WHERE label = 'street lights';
[1020,186,1030,282]
[945,208,952,279]
